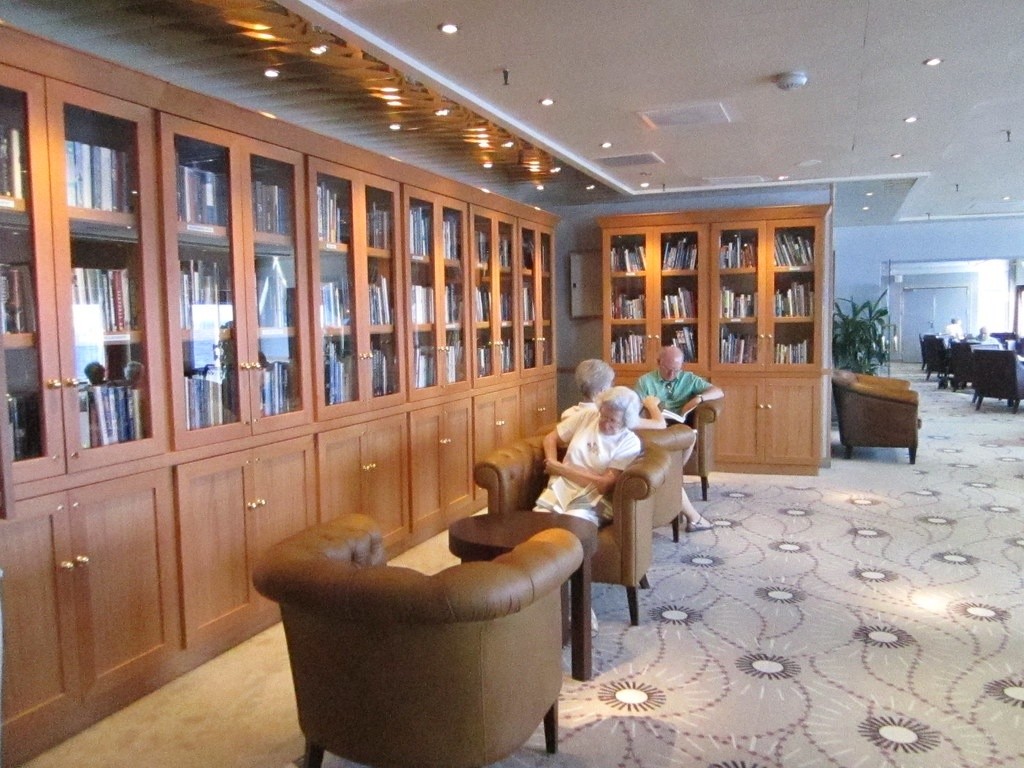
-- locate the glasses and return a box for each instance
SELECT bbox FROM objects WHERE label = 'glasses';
[660,361,681,373]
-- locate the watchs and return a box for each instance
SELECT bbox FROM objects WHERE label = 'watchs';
[696,394,705,404]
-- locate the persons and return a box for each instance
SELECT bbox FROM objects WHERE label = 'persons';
[975,326,1002,350]
[532,386,641,638]
[946,317,963,340]
[561,359,716,532]
[633,346,725,467]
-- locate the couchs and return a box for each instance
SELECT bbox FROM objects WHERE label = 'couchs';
[534,424,698,542]
[919,332,1024,414]
[830,370,923,465]
[474,434,672,628]
[681,400,722,502]
[251,513,583,768]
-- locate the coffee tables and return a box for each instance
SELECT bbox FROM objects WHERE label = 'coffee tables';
[447,508,605,681]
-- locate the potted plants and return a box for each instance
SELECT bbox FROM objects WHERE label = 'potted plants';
[831,289,897,423]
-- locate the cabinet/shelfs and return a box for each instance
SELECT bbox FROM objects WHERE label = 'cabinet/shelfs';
[0,25,561,768]
[596,203,833,477]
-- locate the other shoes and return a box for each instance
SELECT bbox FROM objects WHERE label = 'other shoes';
[569,607,598,637]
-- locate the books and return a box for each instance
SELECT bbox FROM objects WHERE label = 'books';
[476,231,548,378]
[536,475,604,513]
[0,126,142,461]
[611,242,646,362]
[639,400,666,420]
[661,406,696,423]
[721,231,757,364]
[662,235,698,363]
[410,205,463,389]
[175,149,297,432]
[316,180,398,405]
[774,231,815,364]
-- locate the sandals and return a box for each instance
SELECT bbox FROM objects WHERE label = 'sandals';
[685,514,714,532]
